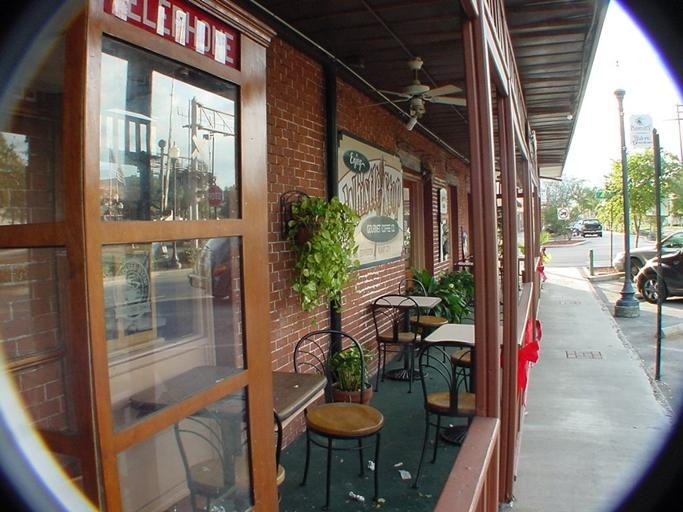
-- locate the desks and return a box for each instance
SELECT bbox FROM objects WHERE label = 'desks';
[371,296,442,380]
[129,366,328,457]
[423,324,503,445]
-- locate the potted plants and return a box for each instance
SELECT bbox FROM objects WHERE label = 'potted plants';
[323,343,376,405]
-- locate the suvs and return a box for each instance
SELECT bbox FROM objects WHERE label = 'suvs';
[574,219,602,238]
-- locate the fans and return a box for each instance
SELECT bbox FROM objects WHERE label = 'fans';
[354,57,467,107]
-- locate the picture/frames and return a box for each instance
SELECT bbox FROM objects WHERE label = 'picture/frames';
[337,133,404,271]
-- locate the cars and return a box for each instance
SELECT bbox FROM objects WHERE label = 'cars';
[635,250,683,303]
[187,236,232,298]
[612,232,683,277]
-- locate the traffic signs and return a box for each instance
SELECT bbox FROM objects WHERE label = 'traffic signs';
[557,207,570,220]
[597,192,615,199]
[629,115,653,150]
[653,150,653,153]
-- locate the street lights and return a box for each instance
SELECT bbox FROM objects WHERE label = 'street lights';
[615,89,639,318]
[167,142,182,268]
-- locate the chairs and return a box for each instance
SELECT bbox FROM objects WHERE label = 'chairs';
[372,294,421,393]
[173,394,286,512]
[450,300,475,393]
[397,279,448,365]
[293,329,384,507]
[411,340,477,489]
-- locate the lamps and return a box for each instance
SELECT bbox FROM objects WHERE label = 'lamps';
[405,99,426,131]
